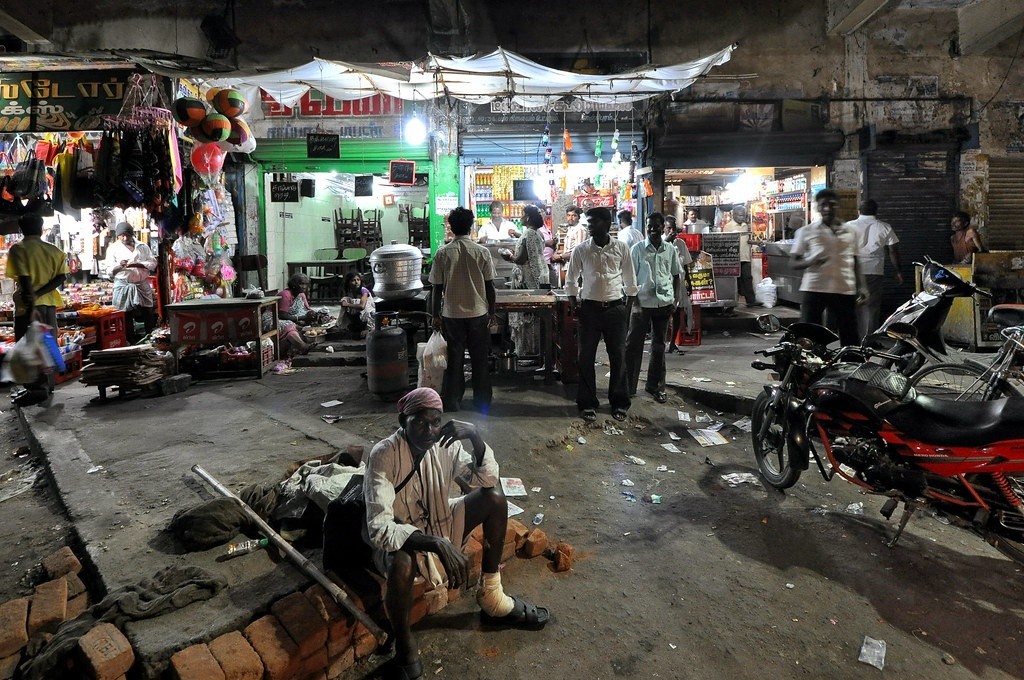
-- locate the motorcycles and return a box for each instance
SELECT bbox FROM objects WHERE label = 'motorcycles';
[749,314,1023,566]
[862,254,1023,381]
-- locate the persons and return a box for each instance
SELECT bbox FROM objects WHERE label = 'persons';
[787,188,869,347]
[535,204,558,289]
[626,213,684,403]
[550,205,588,287]
[275,272,315,325]
[262,309,318,355]
[105,222,157,345]
[564,207,642,421]
[478,201,518,242]
[950,211,985,265]
[661,215,693,354]
[428,207,498,412]
[501,204,549,357]
[6,214,70,406]
[847,199,903,334]
[361,387,549,680]
[724,205,764,308]
[616,210,644,248]
[685,209,705,224]
[336,272,375,332]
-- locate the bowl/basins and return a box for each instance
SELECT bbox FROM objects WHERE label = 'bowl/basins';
[303,332,328,344]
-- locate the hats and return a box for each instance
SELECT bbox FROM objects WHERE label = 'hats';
[117,222,133,236]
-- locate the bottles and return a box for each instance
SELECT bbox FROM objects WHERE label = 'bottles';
[594,135,603,186]
[226,539,268,559]
[620,178,654,199]
[766,174,806,210]
[611,128,620,167]
[542,125,554,185]
[561,129,572,190]
[475,172,551,233]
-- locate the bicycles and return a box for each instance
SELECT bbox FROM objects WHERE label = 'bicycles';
[908,325,1024,403]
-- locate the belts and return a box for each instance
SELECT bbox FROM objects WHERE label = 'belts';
[582,298,623,308]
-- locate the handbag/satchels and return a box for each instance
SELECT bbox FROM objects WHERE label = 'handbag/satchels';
[423,330,448,371]
[0,132,106,234]
[0,319,66,384]
[323,475,366,586]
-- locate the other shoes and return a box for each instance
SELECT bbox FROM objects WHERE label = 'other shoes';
[747,301,763,307]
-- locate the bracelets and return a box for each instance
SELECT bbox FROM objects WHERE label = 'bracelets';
[560,254,562,258]
[297,316,298,321]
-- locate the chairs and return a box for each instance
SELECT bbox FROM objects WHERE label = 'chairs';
[310,207,430,306]
[227,255,278,297]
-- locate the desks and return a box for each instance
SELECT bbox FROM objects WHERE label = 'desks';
[493,296,556,385]
[165,295,283,379]
[287,259,357,306]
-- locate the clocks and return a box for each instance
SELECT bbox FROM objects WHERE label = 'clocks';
[384,195,394,206]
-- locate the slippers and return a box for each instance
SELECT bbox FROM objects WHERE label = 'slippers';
[395,662,424,680]
[479,595,550,629]
[669,345,685,356]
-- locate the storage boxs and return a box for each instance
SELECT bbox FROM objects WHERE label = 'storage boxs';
[55,312,126,384]
[668,304,701,345]
[552,290,580,384]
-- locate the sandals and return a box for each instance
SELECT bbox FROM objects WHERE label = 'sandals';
[11,388,27,398]
[612,407,627,421]
[652,390,667,402]
[11,392,48,404]
[581,408,597,421]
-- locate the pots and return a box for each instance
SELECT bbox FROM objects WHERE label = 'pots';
[684,223,711,234]
